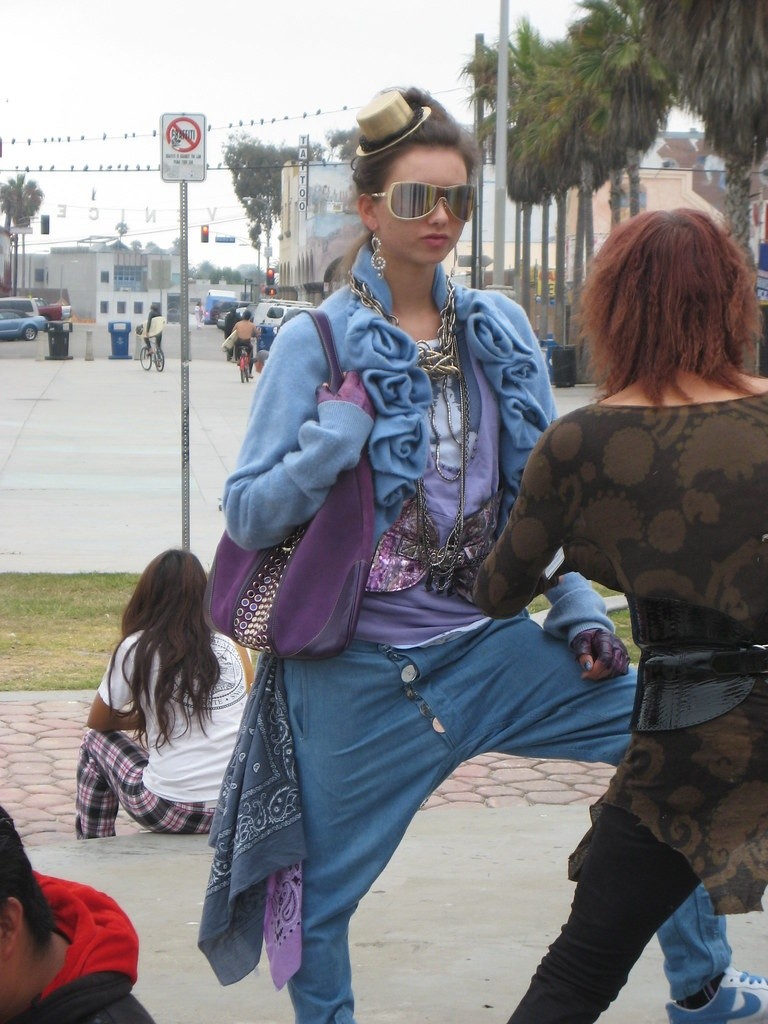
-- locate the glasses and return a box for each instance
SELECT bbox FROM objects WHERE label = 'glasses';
[367,181,477,223]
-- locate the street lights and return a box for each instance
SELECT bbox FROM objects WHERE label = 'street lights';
[245,278,252,302]
[250,284,257,301]
[242,197,269,294]
[60,260,78,302]
[28,250,50,297]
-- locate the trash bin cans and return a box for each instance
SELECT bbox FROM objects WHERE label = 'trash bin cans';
[108,321,133,359]
[44,321,73,360]
[552,344,577,388]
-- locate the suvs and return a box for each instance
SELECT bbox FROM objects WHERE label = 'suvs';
[210,300,317,332]
[0,297,39,316]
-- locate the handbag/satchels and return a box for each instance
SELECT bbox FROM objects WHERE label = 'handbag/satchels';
[206,311,377,659]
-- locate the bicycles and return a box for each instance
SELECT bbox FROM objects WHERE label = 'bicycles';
[141,338,165,372]
[237,345,251,383]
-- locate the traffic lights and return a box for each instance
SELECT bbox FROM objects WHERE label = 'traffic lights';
[266,268,274,286]
[265,288,276,295]
[201,224,208,242]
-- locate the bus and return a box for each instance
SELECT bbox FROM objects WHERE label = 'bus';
[203,289,237,325]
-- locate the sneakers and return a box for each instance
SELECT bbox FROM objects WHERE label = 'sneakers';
[666,964,768,1024]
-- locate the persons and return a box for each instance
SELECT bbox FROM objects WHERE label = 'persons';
[143,303,162,367]
[232,310,260,378]
[75,549,251,841]
[471,209,768,1024]
[224,307,243,361]
[0,805,156,1024]
[221,90,768,1024]
[195,301,203,329]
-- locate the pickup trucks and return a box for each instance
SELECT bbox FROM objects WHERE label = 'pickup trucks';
[35,298,72,323]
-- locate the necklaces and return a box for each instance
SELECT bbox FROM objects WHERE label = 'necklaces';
[346,268,470,589]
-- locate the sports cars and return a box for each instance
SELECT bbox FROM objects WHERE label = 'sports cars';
[0,310,48,341]
